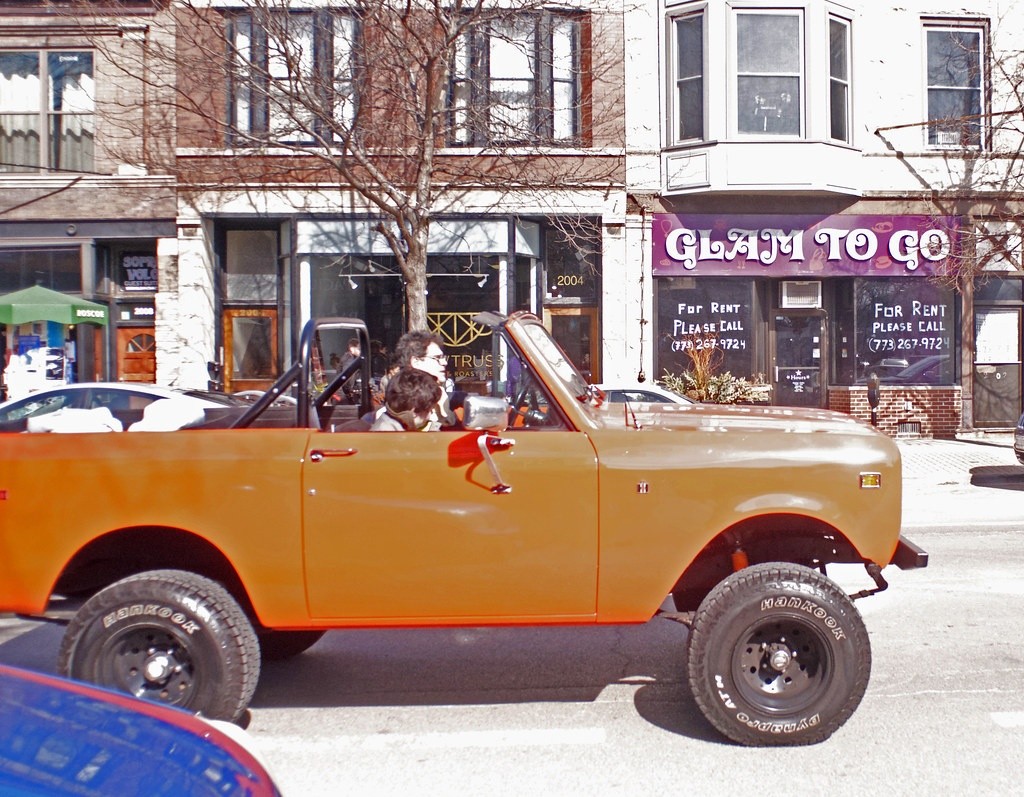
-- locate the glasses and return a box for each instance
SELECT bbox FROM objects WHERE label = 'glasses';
[422,354,449,361]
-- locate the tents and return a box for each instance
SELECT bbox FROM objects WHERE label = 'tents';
[0,284,110,380]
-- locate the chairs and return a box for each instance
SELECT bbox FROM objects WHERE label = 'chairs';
[328,411,378,433]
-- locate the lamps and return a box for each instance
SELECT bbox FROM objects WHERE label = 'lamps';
[551,280,558,290]
[558,291,562,298]
[478,276,487,288]
[369,261,375,272]
[348,276,358,289]
[778,281,822,309]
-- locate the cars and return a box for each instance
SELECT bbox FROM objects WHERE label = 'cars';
[583,380,704,406]
[0,310,932,747]
[838,355,953,384]
[232,391,297,407]
[0,382,256,433]
[1,661,285,796]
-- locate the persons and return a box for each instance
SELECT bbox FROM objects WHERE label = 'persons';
[335,330,521,437]
